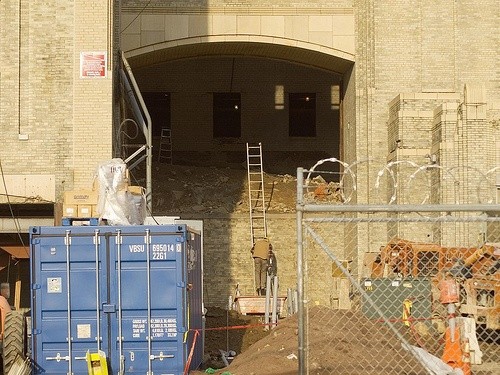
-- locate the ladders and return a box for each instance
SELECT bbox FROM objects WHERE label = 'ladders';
[247,141,271,248]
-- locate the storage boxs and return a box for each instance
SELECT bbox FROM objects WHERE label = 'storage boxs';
[77,204,91,218]
[29,218,205,375]
[65,191,98,204]
[91,204,99,217]
[128,186,143,195]
[63,203,77,217]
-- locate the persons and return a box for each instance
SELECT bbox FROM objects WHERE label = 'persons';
[250,237,272,291]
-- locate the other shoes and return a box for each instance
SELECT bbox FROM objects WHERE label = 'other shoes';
[261,289,264,295]
[256,289,260,291]
